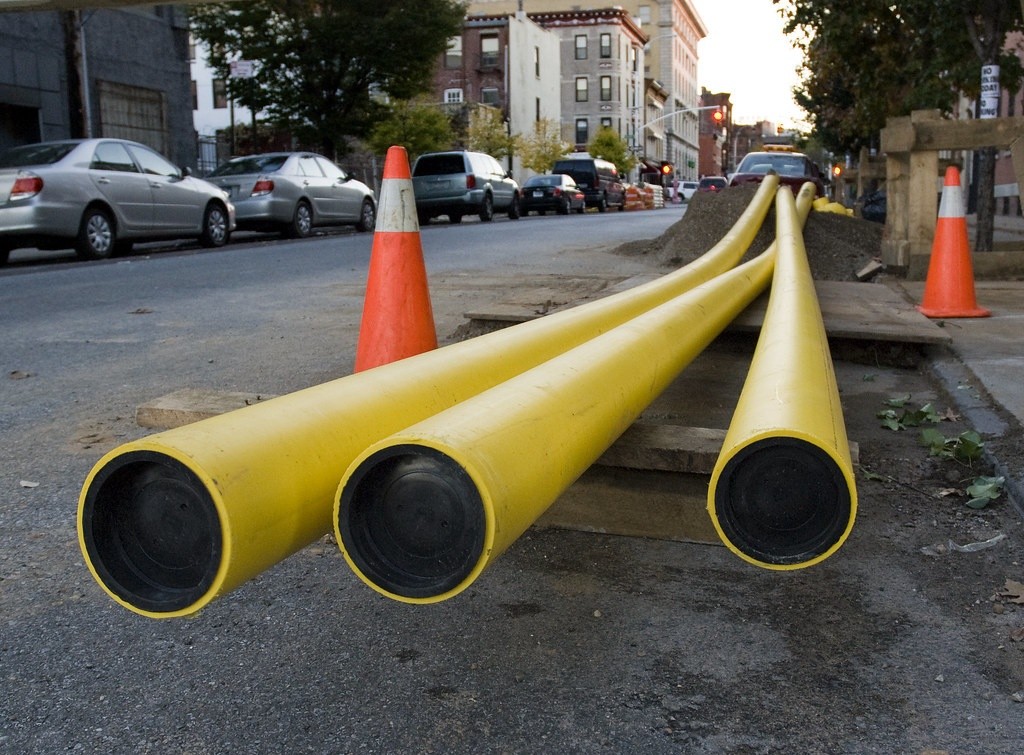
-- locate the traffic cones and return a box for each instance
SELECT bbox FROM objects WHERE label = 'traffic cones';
[352,145,440,374]
[914,166,992,318]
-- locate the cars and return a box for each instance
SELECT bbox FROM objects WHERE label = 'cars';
[0,138,237,265]
[520,175,585,215]
[698,177,727,193]
[728,144,825,196]
[202,152,377,239]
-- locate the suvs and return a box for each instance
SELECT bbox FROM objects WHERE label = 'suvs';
[666,181,700,201]
[412,151,522,224]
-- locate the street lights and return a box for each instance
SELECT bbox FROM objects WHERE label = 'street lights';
[636,34,676,180]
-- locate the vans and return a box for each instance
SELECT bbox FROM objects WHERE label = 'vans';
[553,159,626,212]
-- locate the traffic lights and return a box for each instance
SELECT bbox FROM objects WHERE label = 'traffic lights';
[712,110,723,121]
[661,161,670,174]
